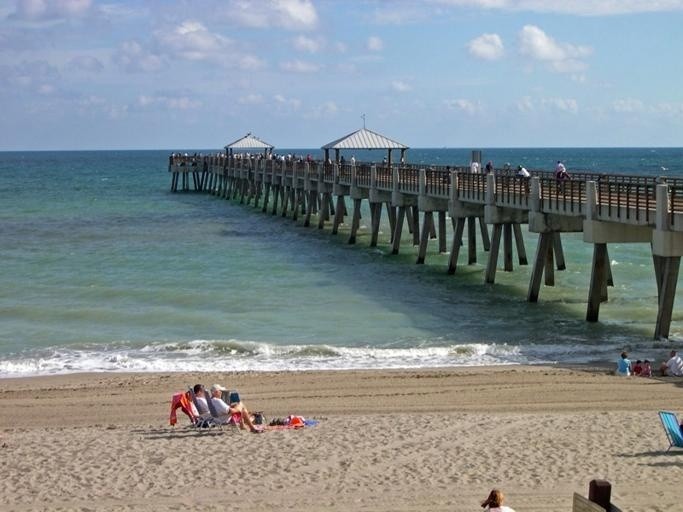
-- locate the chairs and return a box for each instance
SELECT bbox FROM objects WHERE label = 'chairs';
[658,410,683,454]
[185,386,241,435]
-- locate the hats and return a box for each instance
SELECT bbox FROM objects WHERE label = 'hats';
[211,384,226,392]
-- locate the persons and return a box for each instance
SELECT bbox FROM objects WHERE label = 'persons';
[471,160,478,174]
[658,350,683,376]
[516,164,531,192]
[556,160,566,185]
[210,384,263,432]
[192,382,211,415]
[639,360,651,377]
[170,151,297,167]
[306,153,312,161]
[299,152,304,161]
[382,156,388,163]
[350,154,355,177]
[339,156,346,176]
[484,159,492,174]
[480,489,514,512]
[614,352,631,376]
[632,359,642,375]
